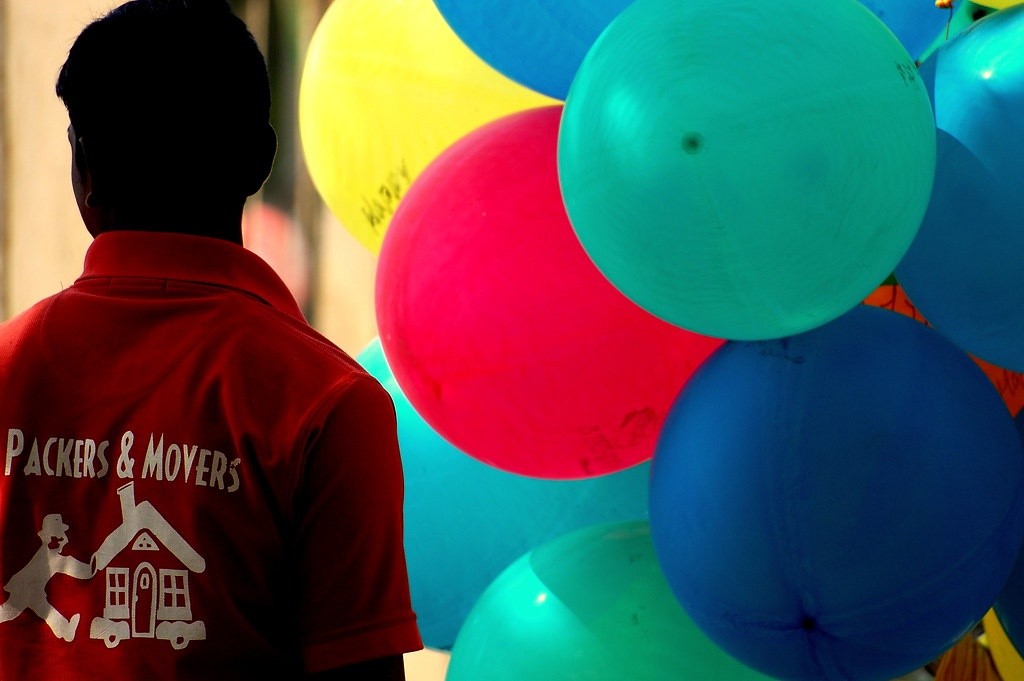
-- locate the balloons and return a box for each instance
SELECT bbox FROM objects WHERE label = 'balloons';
[298,0,1024,681]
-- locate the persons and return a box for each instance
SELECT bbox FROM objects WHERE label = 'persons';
[0,0,423,681]
